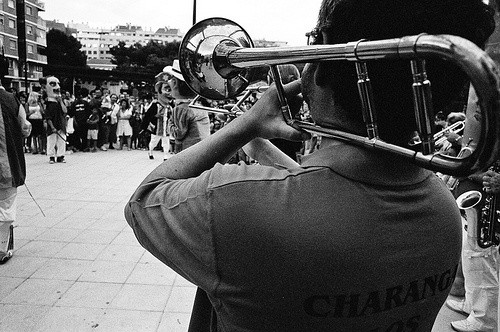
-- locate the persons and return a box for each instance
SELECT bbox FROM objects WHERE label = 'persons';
[418,50,500,332]
[125,0,495,331]
[8,74,322,171]
[1,54,32,265]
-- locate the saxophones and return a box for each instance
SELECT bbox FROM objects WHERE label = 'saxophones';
[455,156,500,254]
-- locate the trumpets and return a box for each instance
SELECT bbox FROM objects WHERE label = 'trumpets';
[433,119,466,147]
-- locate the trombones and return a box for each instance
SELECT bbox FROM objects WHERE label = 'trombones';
[178,18,500,180]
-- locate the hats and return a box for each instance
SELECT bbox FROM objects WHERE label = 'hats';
[163,59,186,82]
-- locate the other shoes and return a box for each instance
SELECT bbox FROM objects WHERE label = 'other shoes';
[50,160,55,164]
[445,300,470,317]
[62,159,67,163]
[451,319,493,332]
